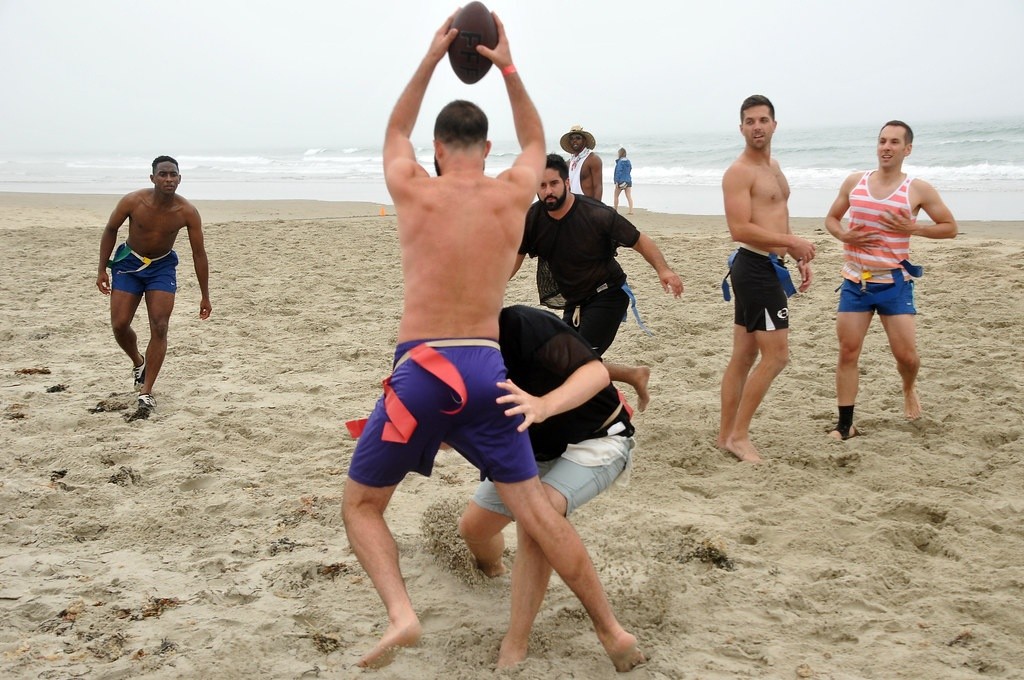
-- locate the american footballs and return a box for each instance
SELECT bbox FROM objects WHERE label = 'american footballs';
[447,0,498,87]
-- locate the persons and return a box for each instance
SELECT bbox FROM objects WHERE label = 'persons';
[452,305,635,673]
[559,123,603,201]
[823,119,959,442]
[613,148,634,215]
[716,94,816,462]
[95,156,212,415]
[508,153,683,413]
[338,5,646,673]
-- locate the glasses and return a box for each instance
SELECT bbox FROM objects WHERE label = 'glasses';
[568,135,583,141]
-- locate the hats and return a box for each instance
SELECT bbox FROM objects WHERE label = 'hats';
[560,126,595,155]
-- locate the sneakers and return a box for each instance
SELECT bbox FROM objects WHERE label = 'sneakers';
[132,354,147,392]
[138,394,157,412]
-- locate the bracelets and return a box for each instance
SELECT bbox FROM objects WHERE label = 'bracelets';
[501,64,517,77]
[796,258,802,262]
